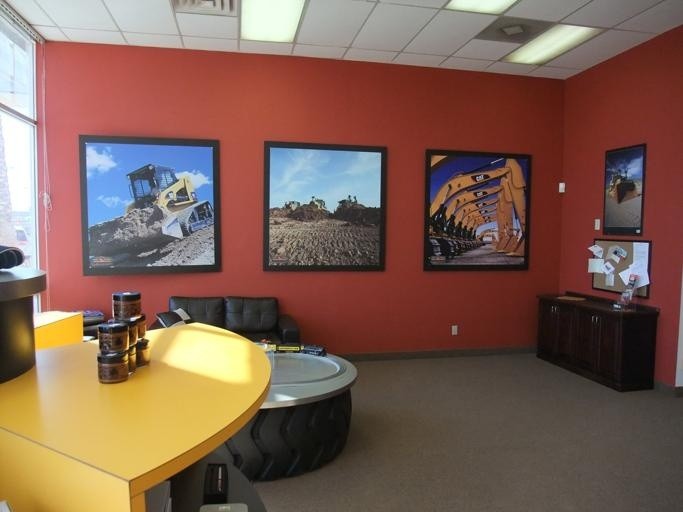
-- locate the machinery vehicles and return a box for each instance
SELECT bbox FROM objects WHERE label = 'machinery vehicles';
[429,154,526,261]
[608,168,639,204]
[88,163,215,257]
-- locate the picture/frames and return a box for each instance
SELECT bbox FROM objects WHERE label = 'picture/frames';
[425,148,528,271]
[603,143,644,234]
[77,134,222,275]
[219,346,359,482]
[592,239,649,297]
[263,140,384,271]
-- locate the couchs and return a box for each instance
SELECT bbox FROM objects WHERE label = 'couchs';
[147,296,303,351]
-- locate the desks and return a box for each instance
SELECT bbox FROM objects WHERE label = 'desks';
[0,312,272,512]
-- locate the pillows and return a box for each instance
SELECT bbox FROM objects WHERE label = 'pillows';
[156,310,191,329]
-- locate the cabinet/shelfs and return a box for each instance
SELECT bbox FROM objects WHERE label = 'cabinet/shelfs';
[536,292,661,392]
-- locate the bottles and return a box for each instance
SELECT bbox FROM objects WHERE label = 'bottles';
[97,323,129,354]
[111,290,142,320]
[107,318,139,347]
[137,313,146,338]
[127,345,137,372]
[96,351,129,384]
[135,338,150,367]
